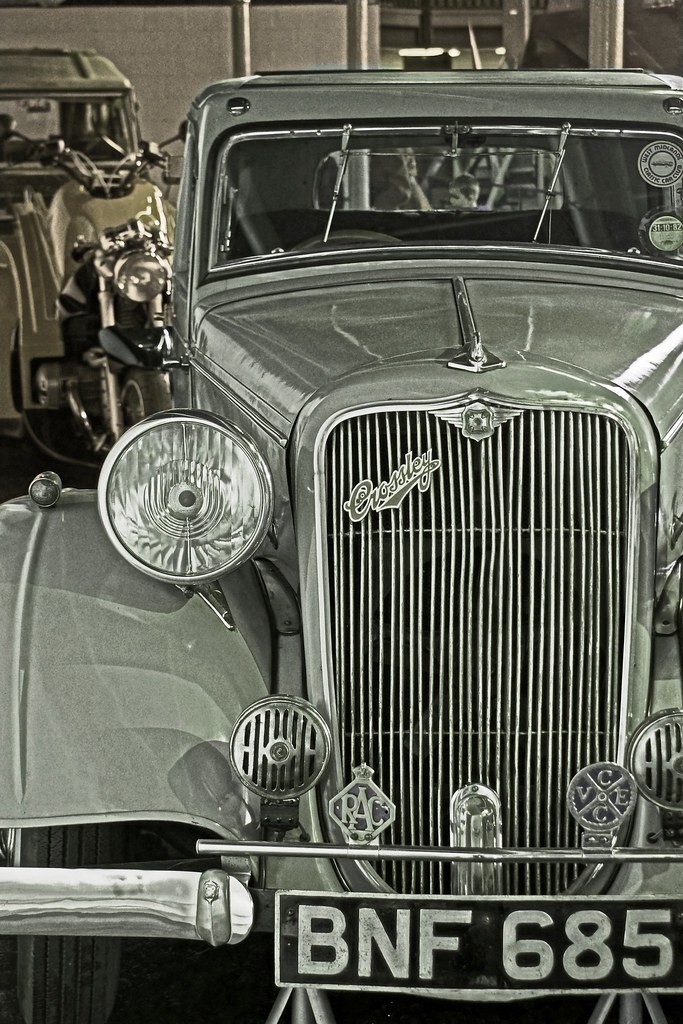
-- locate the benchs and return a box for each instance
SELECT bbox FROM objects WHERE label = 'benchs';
[224,208,651,260]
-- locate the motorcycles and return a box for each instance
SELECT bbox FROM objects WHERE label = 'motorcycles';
[0,111,188,493]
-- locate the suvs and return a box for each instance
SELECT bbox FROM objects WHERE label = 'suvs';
[0,69,683,1023]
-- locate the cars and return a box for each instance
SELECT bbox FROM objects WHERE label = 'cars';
[0,48,184,235]
